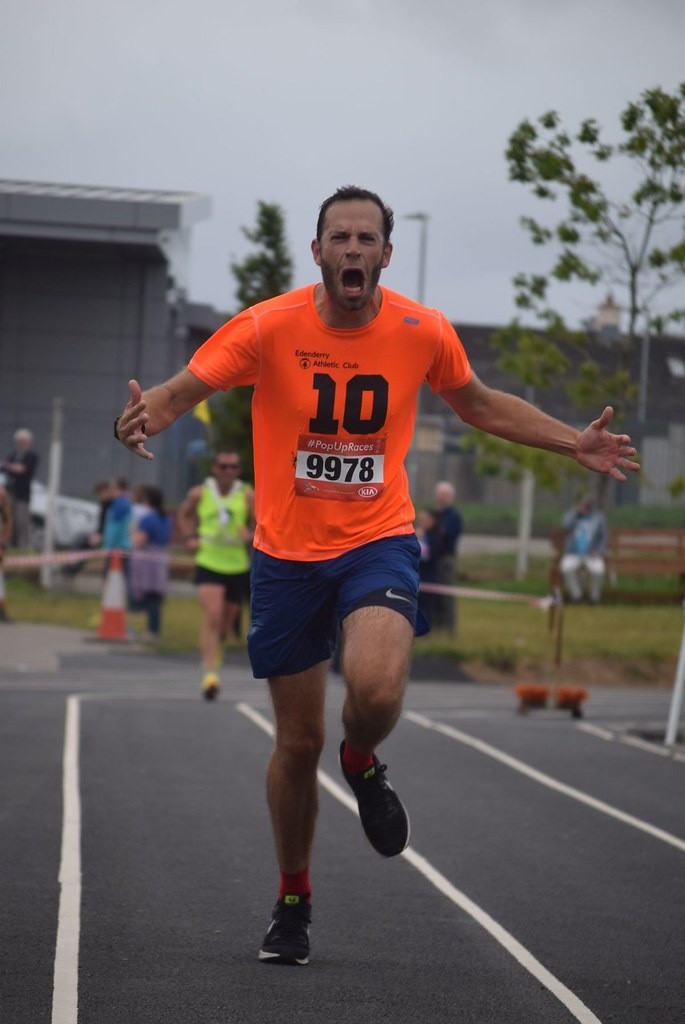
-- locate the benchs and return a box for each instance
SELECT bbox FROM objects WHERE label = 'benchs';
[550,528,684,601]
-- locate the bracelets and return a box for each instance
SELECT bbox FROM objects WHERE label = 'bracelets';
[114,416,145,441]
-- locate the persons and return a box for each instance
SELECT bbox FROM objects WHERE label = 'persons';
[112,187,644,965]
[434,482,461,545]
[179,447,255,700]
[1,428,40,546]
[89,478,172,638]
[561,495,608,602]
[419,507,456,630]
[0,474,14,624]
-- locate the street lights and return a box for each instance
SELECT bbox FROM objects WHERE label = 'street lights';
[403,210,429,307]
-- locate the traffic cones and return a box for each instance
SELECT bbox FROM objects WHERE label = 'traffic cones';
[88,548,136,644]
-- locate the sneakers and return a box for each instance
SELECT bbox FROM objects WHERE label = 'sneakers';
[202,673,219,702]
[338,738,412,859]
[258,891,314,965]
